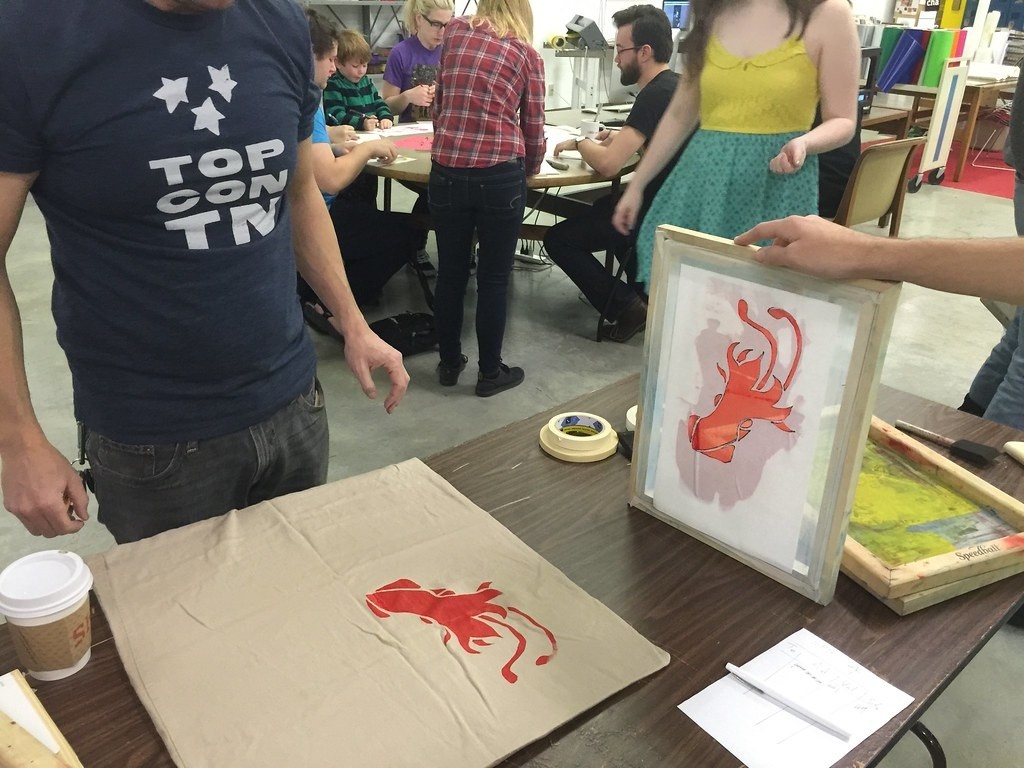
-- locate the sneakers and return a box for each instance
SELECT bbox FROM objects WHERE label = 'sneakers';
[475,367,525,397]
[439,361,465,386]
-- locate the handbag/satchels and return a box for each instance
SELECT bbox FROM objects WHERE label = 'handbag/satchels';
[368,311,438,357]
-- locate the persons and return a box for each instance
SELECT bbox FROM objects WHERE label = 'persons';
[0,0,408,548]
[956,65,1024,432]
[612,0,863,294]
[426,0,545,397]
[542,5,700,344]
[732,213,1024,305]
[382,0,477,277]
[323,29,394,208]
[296,8,420,340]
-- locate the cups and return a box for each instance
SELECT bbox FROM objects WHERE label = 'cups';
[581,119,607,138]
[0,548,93,681]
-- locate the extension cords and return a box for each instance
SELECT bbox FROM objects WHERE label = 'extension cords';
[515,251,547,264]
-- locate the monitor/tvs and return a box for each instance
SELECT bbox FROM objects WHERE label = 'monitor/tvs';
[663,0,692,31]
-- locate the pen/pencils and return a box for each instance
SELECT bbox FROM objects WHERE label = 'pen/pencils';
[328,113,340,126]
[725,662,851,739]
[414,77,423,87]
[349,109,382,124]
[893,420,1001,465]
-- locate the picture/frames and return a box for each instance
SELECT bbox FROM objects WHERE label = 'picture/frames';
[624,225,1024,615]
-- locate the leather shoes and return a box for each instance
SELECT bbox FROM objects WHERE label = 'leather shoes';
[602,291,649,342]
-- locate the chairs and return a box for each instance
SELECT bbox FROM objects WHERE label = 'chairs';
[971,96,1018,173]
[830,137,929,240]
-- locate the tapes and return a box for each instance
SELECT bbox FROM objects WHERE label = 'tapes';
[626,404,638,432]
[547,410,612,450]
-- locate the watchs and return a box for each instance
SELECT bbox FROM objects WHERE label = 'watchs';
[575,135,590,150]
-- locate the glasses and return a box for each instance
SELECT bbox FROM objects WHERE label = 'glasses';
[617,45,640,57]
[421,14,448,29]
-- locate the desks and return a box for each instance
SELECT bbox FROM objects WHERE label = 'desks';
[330,118,643,341]
[0,364,1024,768]
[860,69,1017,183]
